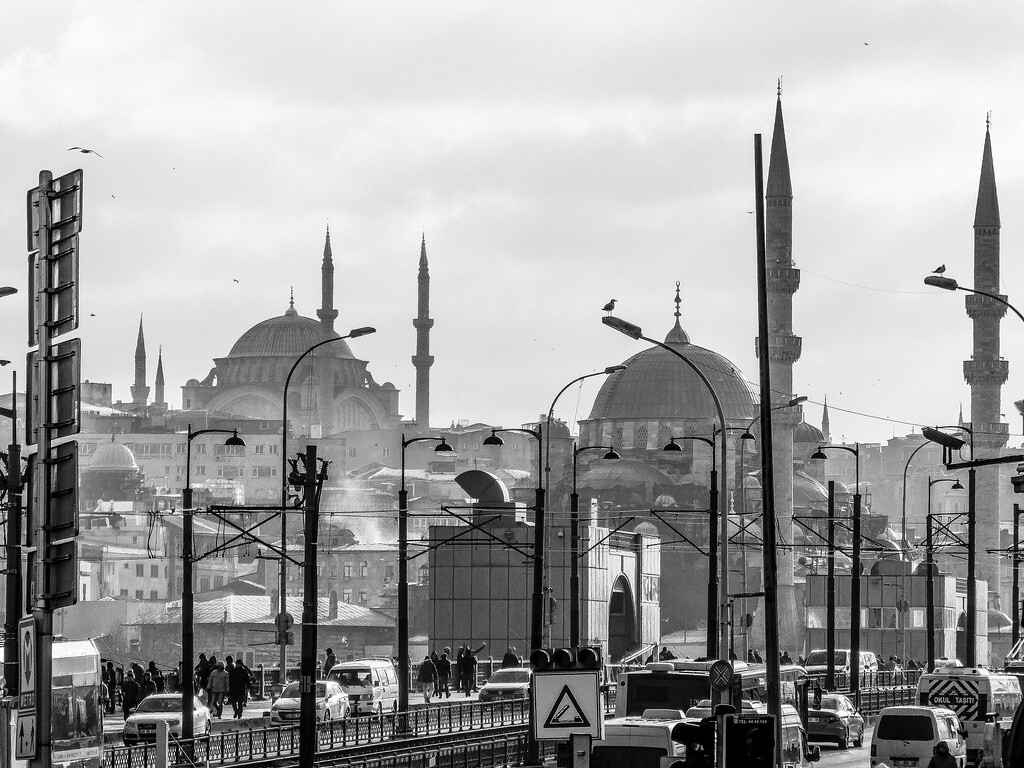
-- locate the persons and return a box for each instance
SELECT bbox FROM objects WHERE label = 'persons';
[502,647,519,669]
[621,647,676,672]
[102,660,165,723]
[779,651,805,667]
[194,653,255,719]
[324,648,337,679]
[875,653,928,684]
[729,648,763,664]
[419,642,486,703]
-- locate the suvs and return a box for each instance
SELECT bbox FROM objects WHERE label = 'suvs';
[804,647,880,690]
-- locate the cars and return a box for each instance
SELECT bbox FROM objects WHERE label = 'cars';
[121,693,214,747]
[270,680,352,730]
[807,693,865,751]
[478,667,532,710]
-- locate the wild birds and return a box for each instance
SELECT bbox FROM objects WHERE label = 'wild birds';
[66,146,104,161]
[90,166,239,318]
[933,264,946,277]
[601,299,618,317]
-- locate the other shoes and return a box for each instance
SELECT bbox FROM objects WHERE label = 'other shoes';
[439,695,442,698]
[238,714,242,719]
[216,710,221,719]
[425,697,430,703]
[234,710,238,718]
[446,693,451,698]
[243,703,246,707]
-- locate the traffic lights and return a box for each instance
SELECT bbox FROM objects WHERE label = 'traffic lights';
[667,718,716,768]
[723,712,777,768]
[528,647,605,672]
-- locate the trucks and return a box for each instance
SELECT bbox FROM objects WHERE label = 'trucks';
[913,662,1024,761]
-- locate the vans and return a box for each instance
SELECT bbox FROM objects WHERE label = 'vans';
[326,657,399,722]
[589,708,703,768]
[869,705,970,768]
[684,697,821,768]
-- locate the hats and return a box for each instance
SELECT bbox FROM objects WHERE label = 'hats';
[216,662,224,667]
[128,674,133,679]
[236,659,242,665]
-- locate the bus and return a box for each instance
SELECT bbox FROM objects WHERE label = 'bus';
[613,659,824,732]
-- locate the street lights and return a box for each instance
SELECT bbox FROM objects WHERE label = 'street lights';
[740,393,810,664]
[599,315,731,703]
[543,363,629,649]
[178,423,247,762]
[899,430,964,669]
[570,440,624,647]
[924,475,967,674]
[661,424,757,660]
[396,432,459,736]
[278,323,381,694]
[483,419,546,654]
